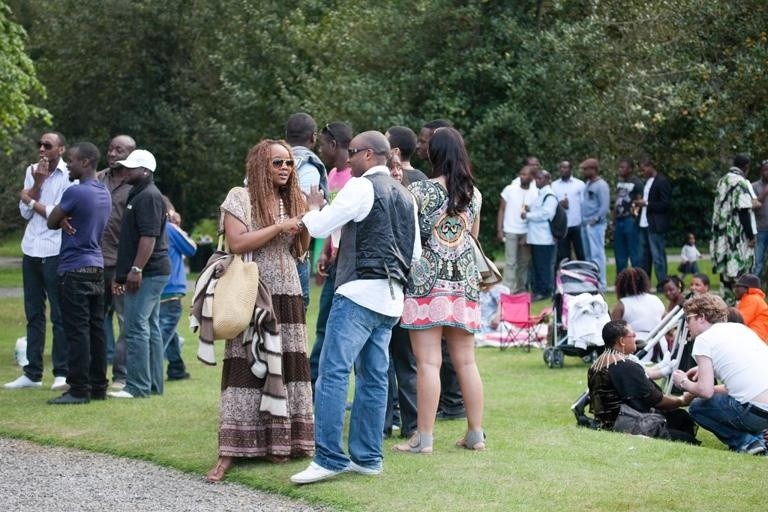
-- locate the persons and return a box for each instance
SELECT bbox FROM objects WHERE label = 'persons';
[709,156,766,307]
[587,266,767,457]
[5,133,197,403]
[499,156,672,293]
[203,112,485,481]
[677,232,702,279]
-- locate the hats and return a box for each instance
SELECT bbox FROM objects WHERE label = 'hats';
[115,149,158,174]
[580,158,599,170]
[732,272,761,289]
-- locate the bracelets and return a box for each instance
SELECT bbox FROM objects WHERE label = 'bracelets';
[679,379,688,390]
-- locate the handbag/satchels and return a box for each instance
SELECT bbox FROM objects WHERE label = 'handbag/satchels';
[613,402,672,442]
[206,187,260,340]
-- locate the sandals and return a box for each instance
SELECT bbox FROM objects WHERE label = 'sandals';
[207,454,233,483]
[457,429,488,448]
[391,430,432,456]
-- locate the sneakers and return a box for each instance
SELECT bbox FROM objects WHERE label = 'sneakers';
[341,458,384,475]
[289,456,350,484]
[108,377,134,399]
[436,409,466,421]
[747,437,767,456]
[3,374,43,390]
[167,370,191,381]
[47,390,91,405]
[51,376,70,391]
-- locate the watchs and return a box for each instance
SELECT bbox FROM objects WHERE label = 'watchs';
[131,267,142,274]
[28,199,36,210]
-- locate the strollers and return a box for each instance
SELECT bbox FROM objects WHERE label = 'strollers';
[570,290,696,440]
[541,257,609,368]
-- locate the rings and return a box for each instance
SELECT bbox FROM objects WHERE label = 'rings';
[297,221,303,228]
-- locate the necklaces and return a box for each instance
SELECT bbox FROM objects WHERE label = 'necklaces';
[273,198,285,236]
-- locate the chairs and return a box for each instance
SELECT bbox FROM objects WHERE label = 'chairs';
[496,291,547,355]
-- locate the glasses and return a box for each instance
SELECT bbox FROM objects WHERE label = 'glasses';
[36,141,53,150]
[347,147,368,157]
[684,313,699,323]
[271,156,295,169]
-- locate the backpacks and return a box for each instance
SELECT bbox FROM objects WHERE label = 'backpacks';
[542,193,570,239]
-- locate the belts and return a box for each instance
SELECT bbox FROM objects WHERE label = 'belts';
[70,265,105,275]
[743,401,768,418]
